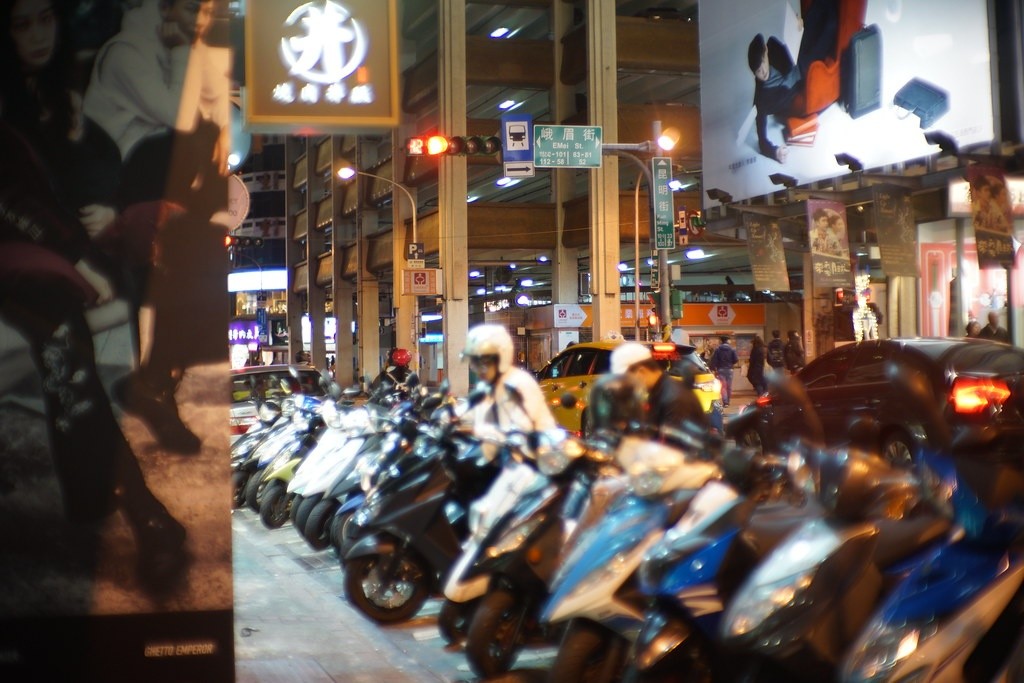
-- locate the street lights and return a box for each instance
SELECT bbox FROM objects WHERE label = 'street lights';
[338,167,421,379]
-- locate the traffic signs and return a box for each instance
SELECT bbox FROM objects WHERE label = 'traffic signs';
[408,242,424,260]
[651,156,676,250]
[553,303,588,328]
[533,124,602,168]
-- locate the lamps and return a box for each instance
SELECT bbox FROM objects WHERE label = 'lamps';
[924,131,959,157]
[835,152,864,174]
[770,173,797,190]
[705,188,732,207]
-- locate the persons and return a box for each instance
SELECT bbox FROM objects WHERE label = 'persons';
[809,208,845,256]
[979,311,1008,342]
[972,176,1009,232]
[748,0,838,163]
[295,351,312,364]
[710,336,737,408]
[766,329,785,373]
[725,276,734,300]
[609,342,713,452]
[785,329,804,373]
[965,322,981,338]
[746,335,769,396]
[331,356,335,379]
[451,321,588,484]
[0,0,235,578]
[369,348,412,390]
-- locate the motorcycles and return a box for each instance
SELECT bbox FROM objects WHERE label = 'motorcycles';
[229,364,1023,683]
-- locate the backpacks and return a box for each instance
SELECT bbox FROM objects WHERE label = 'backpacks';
[768,341,783,364]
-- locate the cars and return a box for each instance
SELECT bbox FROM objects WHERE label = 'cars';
[536,338,726,440]
[229,362,332,447]
[724,335,1023,483]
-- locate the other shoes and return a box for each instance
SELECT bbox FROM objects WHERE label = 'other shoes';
[112,370,205,455]
[723,403,728,407]
[135,511,189,596]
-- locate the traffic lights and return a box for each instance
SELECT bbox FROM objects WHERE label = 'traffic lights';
[403,134,504,158]
[225,235,264,249]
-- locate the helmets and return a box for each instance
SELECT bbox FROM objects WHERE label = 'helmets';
[392,349,412,366]
[462,325,515,373]
[611,343,652,374]
